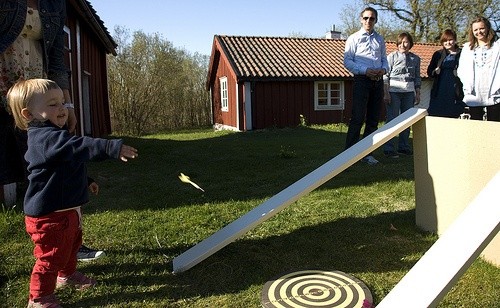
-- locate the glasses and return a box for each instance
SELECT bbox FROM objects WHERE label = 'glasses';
[363,17,375,21]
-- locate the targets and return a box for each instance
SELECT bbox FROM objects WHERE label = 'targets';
[259,267,374,308]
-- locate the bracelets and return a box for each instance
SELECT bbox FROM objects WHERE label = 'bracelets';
[64,103,74,108]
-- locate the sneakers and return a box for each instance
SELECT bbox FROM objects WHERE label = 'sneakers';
[27,294,62,308]
[76,245,105,262]
[56,270,98,291]
[384,151,414,159]
[360,155,379,165]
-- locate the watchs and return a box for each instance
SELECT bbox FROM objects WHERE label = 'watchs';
[383,68,386,74]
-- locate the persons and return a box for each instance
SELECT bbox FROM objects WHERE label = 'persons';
[6,78,138,308]
[427,29,464,118]
[0,0,106,262]
[383,30,421,158]
[345,7,390,165]
[457,15,500,122]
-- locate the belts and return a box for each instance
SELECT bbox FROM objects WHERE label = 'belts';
[371,76,381,81]
